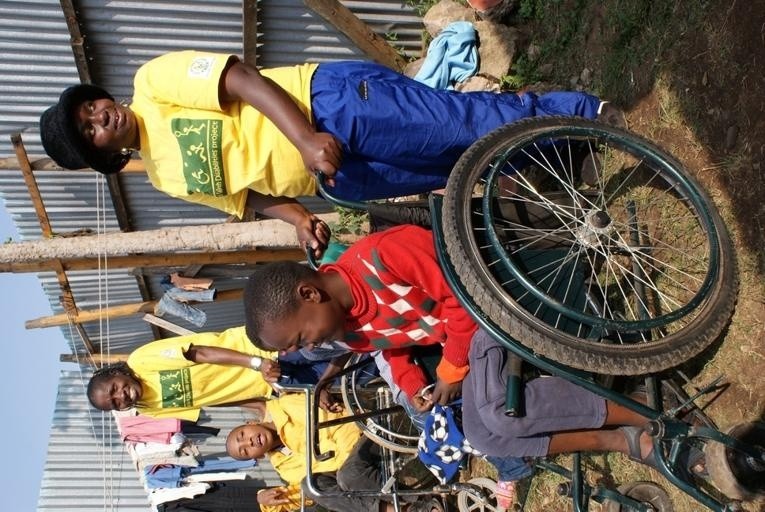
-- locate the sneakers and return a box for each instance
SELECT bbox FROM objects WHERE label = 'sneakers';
[594,101,629,136]
[570,146,604,188]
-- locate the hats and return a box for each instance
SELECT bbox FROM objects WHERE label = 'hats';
[39,84,131,173]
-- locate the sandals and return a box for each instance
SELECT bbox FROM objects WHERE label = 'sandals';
[627,373,719,436]
[617,425,707,482]
[399,495,444,512]
